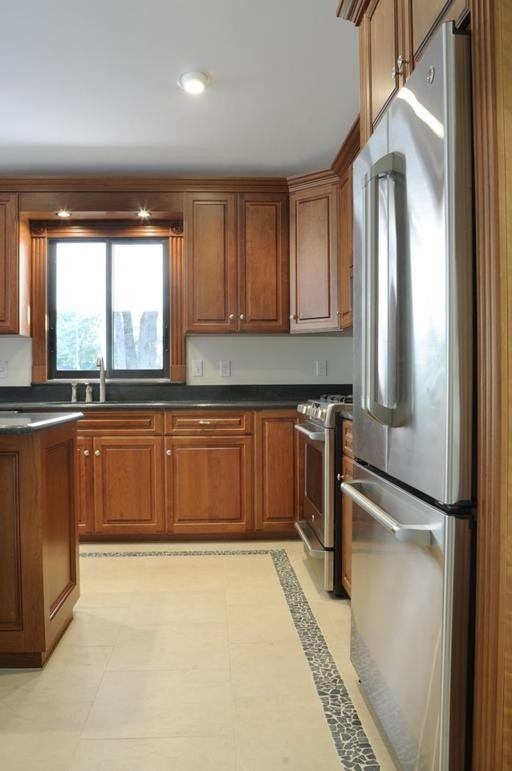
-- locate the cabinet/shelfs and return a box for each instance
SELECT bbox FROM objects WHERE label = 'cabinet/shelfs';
[78,410,305,544]
[290,162,351,336]
[336,1,511,153]
[0,175,33,338]
[181,179,291,337]
[1,419,83,669]
[341,418,357,601]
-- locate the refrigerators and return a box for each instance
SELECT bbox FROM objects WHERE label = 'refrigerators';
[343,18,477,771]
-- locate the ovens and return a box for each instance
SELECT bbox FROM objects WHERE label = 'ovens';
[287,419,343,594]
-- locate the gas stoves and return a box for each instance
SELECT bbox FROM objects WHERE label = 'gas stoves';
[295,390,353,430]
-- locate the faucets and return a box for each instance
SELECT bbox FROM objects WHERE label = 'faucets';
[96,355,106,402]
[86,386,94,402]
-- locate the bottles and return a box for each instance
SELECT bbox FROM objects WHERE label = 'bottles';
[84,382,93,403]
[71,382,78,402]
